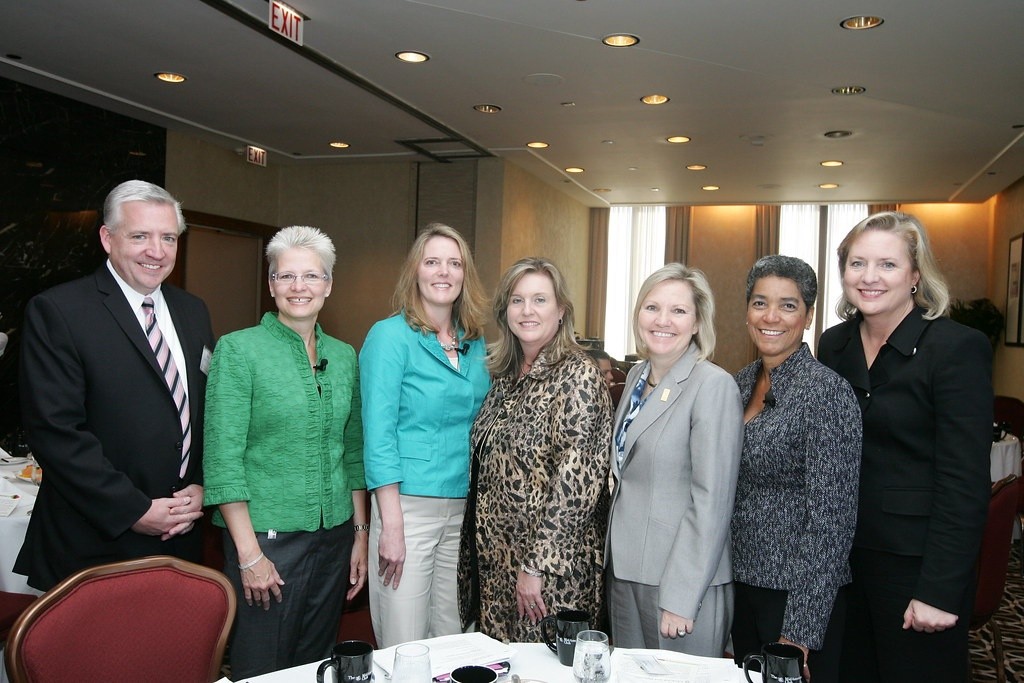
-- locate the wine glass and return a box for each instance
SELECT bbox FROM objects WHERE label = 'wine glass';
[28,459,44,515]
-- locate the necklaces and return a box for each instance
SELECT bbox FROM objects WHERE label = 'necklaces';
[521,356,526,375]
[439,330,456,351]
[646,378,656,387]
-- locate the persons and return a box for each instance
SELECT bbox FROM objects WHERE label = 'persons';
[817,211,995,683]
[357,223,492,651]
[456,256,614,643]
[591,349,614,386]
[731,253,862,683]
[11,179,213,592]
[204,224,369,681]
[604,263,742,658]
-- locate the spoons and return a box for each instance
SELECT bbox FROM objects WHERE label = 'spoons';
[373,660,392,680]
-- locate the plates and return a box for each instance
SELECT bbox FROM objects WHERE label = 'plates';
[0,457,28,465]
[15,470,31,482]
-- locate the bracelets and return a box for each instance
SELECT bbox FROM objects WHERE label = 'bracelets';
[238,552,264,569]
[521,563,545,577]
[353,524,369,533]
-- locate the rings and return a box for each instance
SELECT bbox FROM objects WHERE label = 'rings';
[678,629,686,635]
[528,603,536,609]
[804,664,807,667]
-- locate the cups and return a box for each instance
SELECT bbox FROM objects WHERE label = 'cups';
[450,664,499,683]
[742,642,806,683]
[540,610,591,666]
[573,630,612,683]
[392,643,433,683]
[502,679,547,683]
[317,640,375,683]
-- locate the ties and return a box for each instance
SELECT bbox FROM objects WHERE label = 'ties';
[142,297,191,479]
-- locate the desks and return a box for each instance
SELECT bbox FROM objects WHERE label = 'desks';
[1,456,45,633]
[245,644,767,683]
[992,428,1022,544]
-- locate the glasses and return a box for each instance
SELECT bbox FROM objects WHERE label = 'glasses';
[270,271,329,283]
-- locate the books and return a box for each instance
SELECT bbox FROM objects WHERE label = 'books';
[434,661,510,683]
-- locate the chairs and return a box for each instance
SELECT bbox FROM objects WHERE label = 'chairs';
[3,556,236,683]
[966,395,1024,683]
